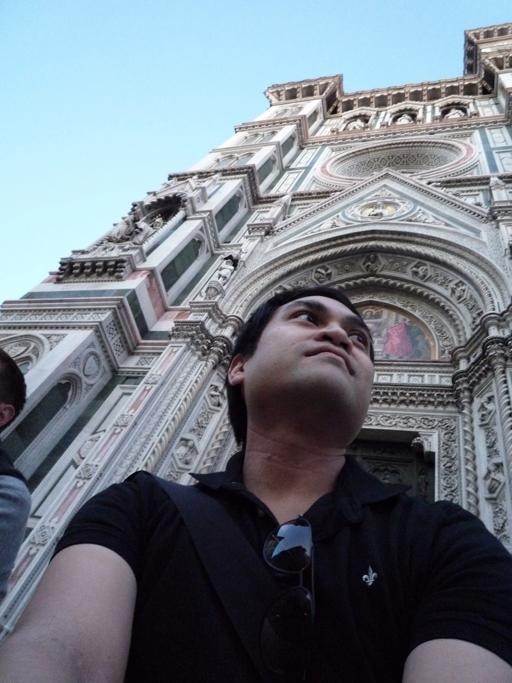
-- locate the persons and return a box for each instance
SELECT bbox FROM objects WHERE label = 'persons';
[0,345,31,606]
[380,315,432,360]
[0,284,512,683]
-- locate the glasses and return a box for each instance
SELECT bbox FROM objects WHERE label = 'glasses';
[259,513,318,680]
